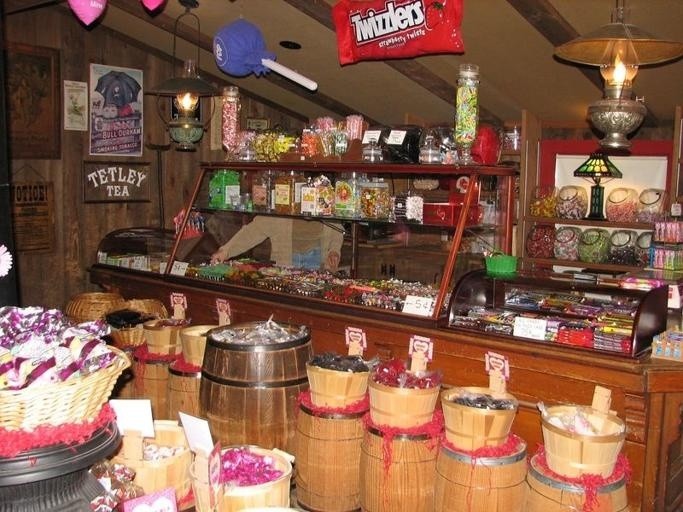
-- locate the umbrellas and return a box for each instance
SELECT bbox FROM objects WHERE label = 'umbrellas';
[94,70,142,108]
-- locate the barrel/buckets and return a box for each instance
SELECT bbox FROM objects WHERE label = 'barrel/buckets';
[111,319,629,511]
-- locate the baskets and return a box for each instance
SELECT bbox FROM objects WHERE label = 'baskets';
[65,292,168,346]
[0,344,132,431]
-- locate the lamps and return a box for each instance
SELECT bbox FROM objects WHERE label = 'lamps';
[553,0,683,149]
[574,152,622,220]
[144,1,220,150]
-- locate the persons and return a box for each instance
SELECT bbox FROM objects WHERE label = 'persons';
[107,82,125,110]
[210,214,345,272]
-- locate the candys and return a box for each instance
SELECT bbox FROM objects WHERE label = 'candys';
[186,257,453,316]
[221,100,295,163]
[526,193,662,266]
[455,79,479,149]
[208,173,389,219]
[302,114,364,157]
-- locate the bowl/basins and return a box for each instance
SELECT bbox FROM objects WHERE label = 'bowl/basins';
[483,255,519,275]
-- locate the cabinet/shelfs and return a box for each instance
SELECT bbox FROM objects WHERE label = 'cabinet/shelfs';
[444,270,668,358]
[515,105,682,283]
[163,160,515,331]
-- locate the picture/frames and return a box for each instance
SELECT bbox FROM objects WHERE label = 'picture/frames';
[6,43,60,160]
[81,160,152,203]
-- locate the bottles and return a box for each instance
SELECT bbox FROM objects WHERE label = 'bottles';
[501,122,520,155]
[240,140,257,163]
[362,137,383,164]
[419,135,442,165]
